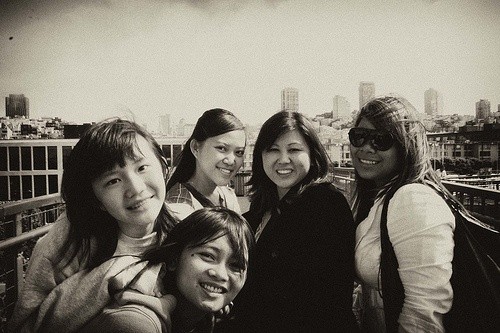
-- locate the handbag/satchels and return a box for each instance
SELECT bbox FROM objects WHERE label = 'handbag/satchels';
[377,179,500,333]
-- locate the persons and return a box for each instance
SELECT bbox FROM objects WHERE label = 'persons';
[6,118,181,333]
[19,206,254,333]
[164,108,246,333]
[436,165,446,178]
[231,111,358,333]
[350,93,457,333]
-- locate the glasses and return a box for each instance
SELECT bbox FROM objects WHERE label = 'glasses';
[348,127,395,151]
